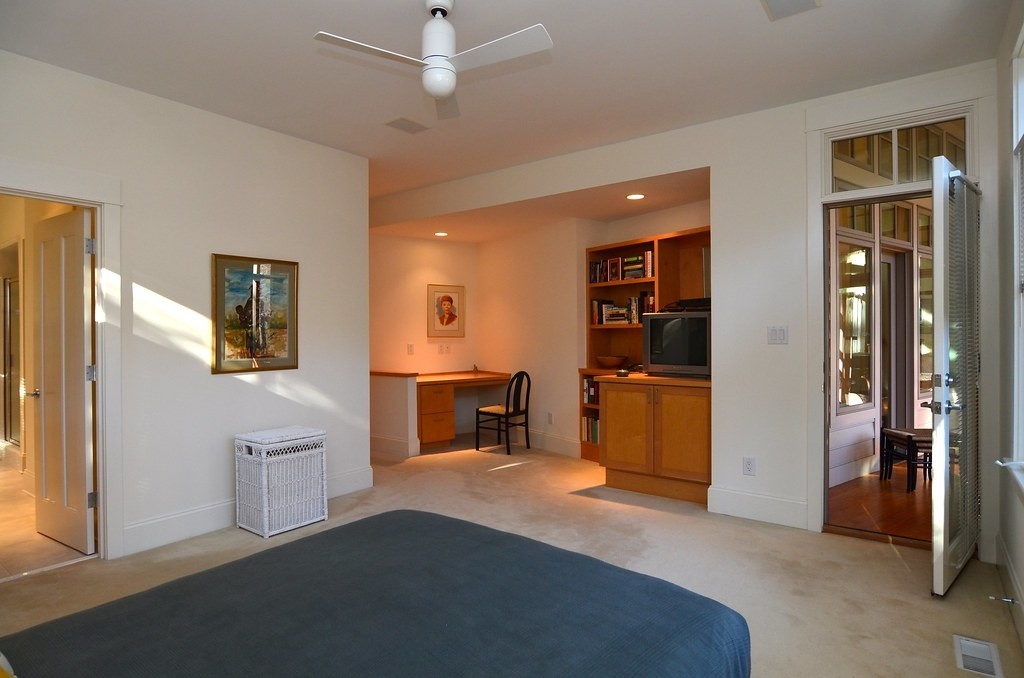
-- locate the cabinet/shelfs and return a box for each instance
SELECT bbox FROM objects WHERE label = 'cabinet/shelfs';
[586,226,710,373]
[579,368,632,466]
[593,374,710,505]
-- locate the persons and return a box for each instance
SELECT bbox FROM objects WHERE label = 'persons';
[439,295,457,326]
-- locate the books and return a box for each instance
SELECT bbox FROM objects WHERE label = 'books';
[590,251,655,283]
[591,290,655,325]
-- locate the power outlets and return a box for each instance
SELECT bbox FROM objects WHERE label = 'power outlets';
[438,344,444,353]
[445,344,451,353]
[408,343,414,355]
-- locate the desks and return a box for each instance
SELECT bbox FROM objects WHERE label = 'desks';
[416,368,511,454]
[235,426,331,538]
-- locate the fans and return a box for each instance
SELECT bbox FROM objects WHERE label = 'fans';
[313,0,555,101]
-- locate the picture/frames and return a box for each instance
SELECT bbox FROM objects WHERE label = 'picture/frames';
[211,255,299,375]
[427,285,465,338]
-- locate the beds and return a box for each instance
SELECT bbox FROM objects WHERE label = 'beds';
[0,508,750,678]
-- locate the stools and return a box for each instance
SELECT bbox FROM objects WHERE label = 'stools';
[879,427,932,490]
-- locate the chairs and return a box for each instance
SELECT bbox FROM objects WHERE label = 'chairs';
[476,370,530,455]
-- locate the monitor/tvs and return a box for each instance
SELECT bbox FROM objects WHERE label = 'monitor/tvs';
[642,311,712,379]
